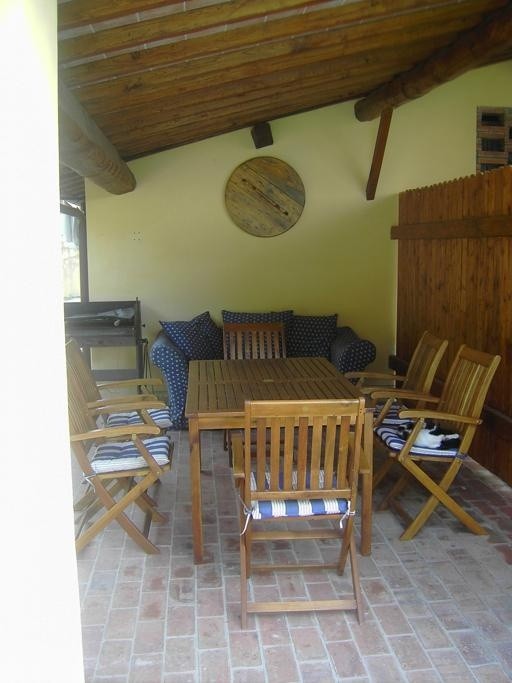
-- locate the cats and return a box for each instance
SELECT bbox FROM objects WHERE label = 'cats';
[397,420,461,450]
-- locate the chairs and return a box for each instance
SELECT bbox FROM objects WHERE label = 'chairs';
[344,330,501,541]
[223,324,287,467]
[232,397,371,631]
[65,338,174,554]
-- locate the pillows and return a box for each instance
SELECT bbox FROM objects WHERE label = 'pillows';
[159,311,224,360]
[288,314,337,357]
[221,310,293,359]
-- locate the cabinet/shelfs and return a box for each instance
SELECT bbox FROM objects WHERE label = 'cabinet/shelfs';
[64,298,163,394]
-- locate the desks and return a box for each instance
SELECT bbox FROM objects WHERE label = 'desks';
[185,358,371,564]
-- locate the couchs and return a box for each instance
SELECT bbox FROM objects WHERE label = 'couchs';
[150,327,376,430]
